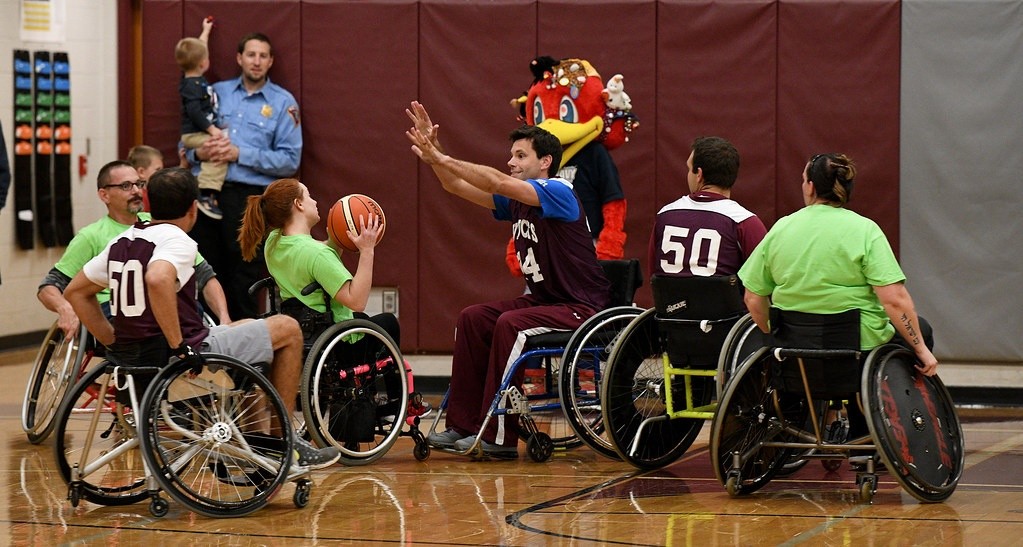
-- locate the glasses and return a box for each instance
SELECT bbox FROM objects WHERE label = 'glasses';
[104,180,144,191]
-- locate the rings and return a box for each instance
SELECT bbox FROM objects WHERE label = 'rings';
[423,143,426,145]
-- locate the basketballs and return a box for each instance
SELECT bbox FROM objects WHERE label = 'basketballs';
[327,191,386,253]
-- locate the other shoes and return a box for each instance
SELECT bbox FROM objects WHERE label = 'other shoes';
[824,421,849,444]
[849,448,876,466]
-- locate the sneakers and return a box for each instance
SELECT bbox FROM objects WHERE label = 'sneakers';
[455,433,519,459]
[428,427,463,446]
[197,196,223,219]
[292,430,341,471]
[418,401,432,419]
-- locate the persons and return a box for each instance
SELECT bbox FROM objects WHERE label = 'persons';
[127,145,189,213]
[647,135,766,277]
[64,169,340,473]
[404,101,602,461]
[238,178,432,420]
[178,31,303,321]
[37,160,272,437]
[175,17,228,220]
[737,154,937,463]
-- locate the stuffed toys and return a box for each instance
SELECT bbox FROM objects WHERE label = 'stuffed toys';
[506,56,639,294]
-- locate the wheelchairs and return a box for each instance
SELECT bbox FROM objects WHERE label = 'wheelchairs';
[247,276,433,467]
[53,325,312,518]
[597,272,826,479]
[418,254,653,465]
[20,301,146,446]
[706,307,965,504]
